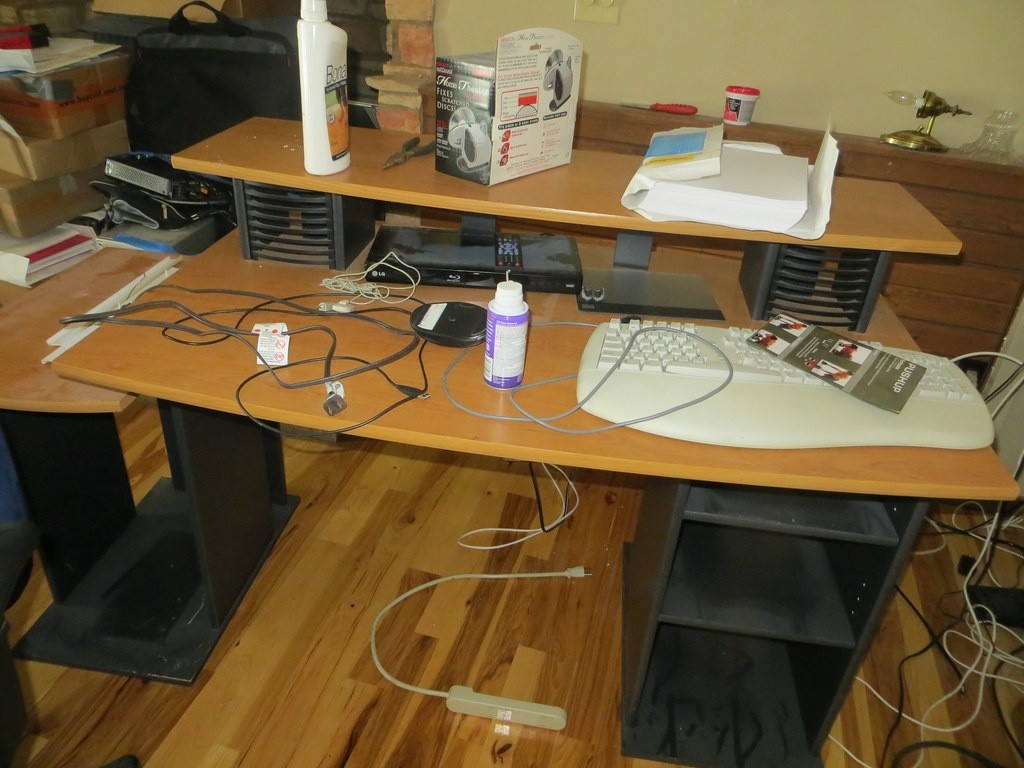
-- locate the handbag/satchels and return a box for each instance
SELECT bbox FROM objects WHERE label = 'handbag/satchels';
[123,2,302,156]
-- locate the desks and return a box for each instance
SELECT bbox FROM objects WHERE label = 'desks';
[0,250,189,606]
[51,118,1024,768]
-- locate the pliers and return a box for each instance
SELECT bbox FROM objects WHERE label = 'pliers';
[382,136,436,170]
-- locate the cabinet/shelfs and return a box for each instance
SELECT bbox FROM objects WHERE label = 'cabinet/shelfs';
[618,479,932,767]
[571,99,1023,401]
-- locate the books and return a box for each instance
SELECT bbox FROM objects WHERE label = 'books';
[0,222,101,289]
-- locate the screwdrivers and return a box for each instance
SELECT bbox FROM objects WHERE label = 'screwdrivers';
[620,102,698,115]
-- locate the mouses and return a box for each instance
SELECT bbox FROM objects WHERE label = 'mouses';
[410,302,487,348]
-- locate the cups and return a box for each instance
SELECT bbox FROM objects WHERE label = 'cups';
[721,86,760,126]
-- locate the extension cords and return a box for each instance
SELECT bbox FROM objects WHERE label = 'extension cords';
[445,684,568,731]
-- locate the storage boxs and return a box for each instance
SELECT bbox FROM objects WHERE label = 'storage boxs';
[126,0,301,153]
[1,2,137,243]
[434,28,586,184]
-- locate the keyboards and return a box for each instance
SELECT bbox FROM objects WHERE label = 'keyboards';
[576,318,996,449]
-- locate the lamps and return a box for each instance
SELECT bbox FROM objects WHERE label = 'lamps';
[880,89,972,153]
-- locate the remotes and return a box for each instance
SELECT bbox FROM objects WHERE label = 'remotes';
[495,232,523,273]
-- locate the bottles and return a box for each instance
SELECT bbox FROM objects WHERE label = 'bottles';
[297,0,352,176]
[483,280,529,391]
